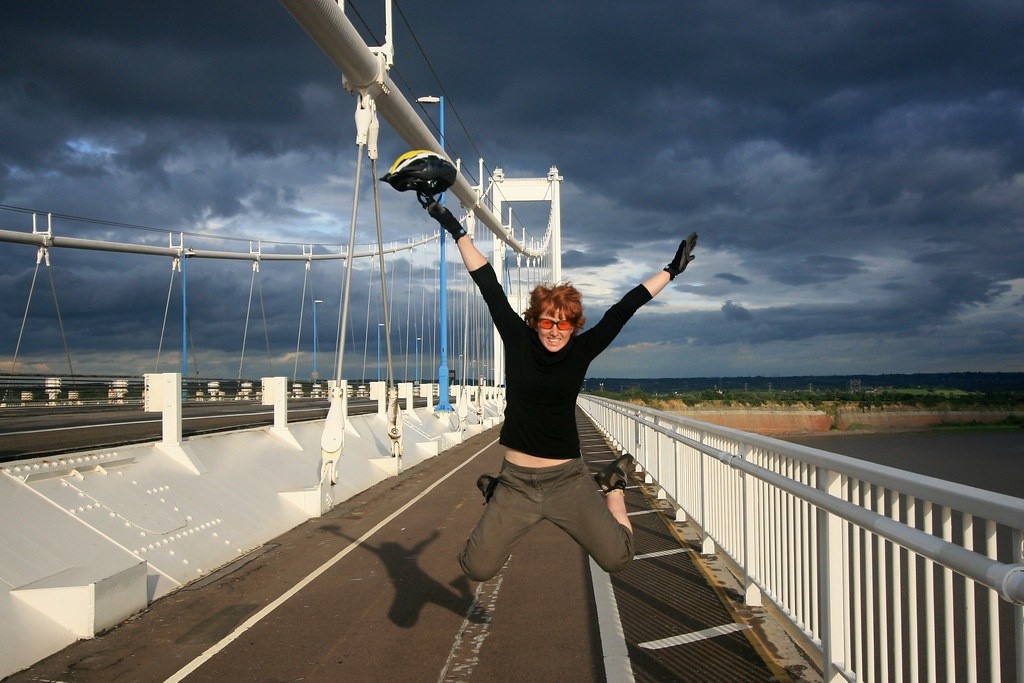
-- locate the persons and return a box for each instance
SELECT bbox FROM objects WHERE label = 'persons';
[426,197,697,582]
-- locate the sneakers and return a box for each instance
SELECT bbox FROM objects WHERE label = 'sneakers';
[477,475,500,498]
[594,453,634,493]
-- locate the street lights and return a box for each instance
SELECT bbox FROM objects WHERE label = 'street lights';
[378,323,385,381]
[312,299,324,385]
[459,354,464,386]
[415,337,422,387]
[414,94,455,413]
[181,249,197,402]
[472,360,477,387]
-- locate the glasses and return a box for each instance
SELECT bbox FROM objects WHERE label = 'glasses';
[534,318,573,330]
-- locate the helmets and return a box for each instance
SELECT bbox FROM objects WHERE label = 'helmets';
[379,149,456,193]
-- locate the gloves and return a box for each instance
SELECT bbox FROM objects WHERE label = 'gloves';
[663,231,697,281]
[426,198,467,243]
